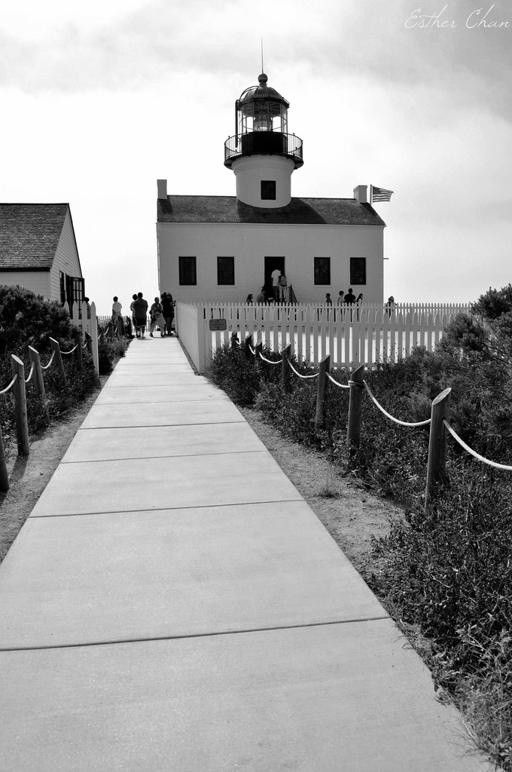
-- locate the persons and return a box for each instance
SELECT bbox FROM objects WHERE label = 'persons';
[324,293,332,306]
[130,293,141,337]
[256,285,270,306]
[344,288,356,304]
[278,270,288,302]
[353,293,365,306]
[385,295,396,318]
[134,292,148,338]
[149,297,167,337]
[160,292,177,336]
[83,296,91,318]
[245,293,253,305]
[337,290,345,305]
[111,296,123,318]
[271,264,282,301]
